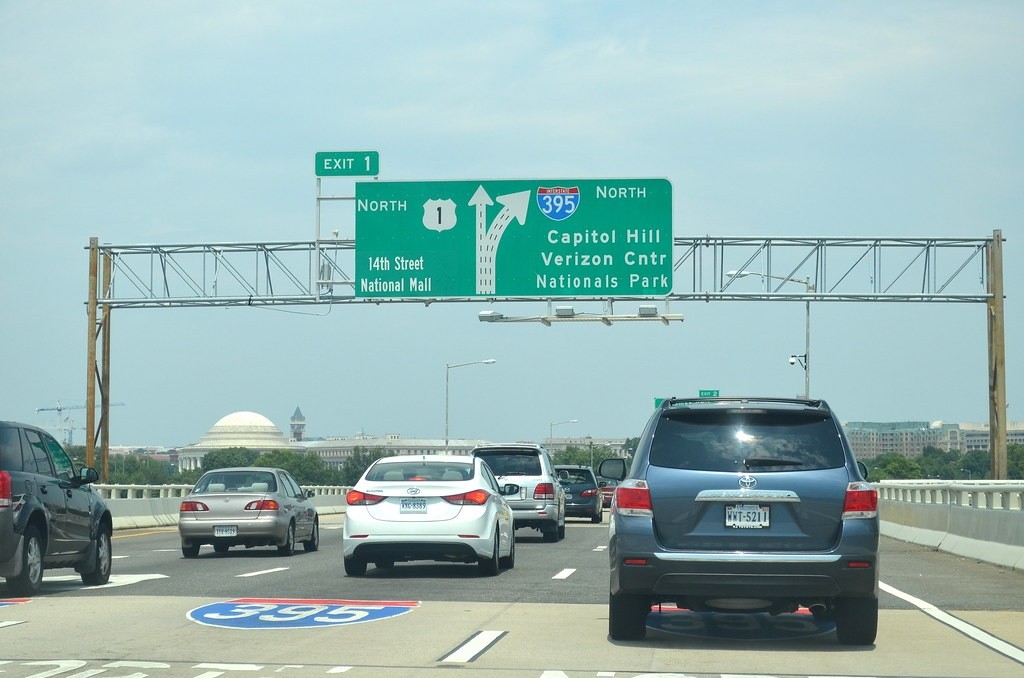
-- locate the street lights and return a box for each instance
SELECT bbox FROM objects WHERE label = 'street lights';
[445,360,497,453]
[550,418,579,448]
[726,270,809,397]
[590,442,611,470]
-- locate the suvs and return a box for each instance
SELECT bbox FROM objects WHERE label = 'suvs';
[471,444,570,542]
[599,398,892,643]
[0,419,115,595]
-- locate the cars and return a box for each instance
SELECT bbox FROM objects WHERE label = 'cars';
[180,467,318,559]
[554,465,609,523]
[595,476,620,505]
[342,456,523,574]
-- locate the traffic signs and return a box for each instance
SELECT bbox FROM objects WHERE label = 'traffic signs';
[355,180,672,299]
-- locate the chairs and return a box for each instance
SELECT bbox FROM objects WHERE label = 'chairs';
[660,440,707,470]
[442,471,463,481]
[252,483,268,492]
[383,471,405,480]
[209,484,225,492]
